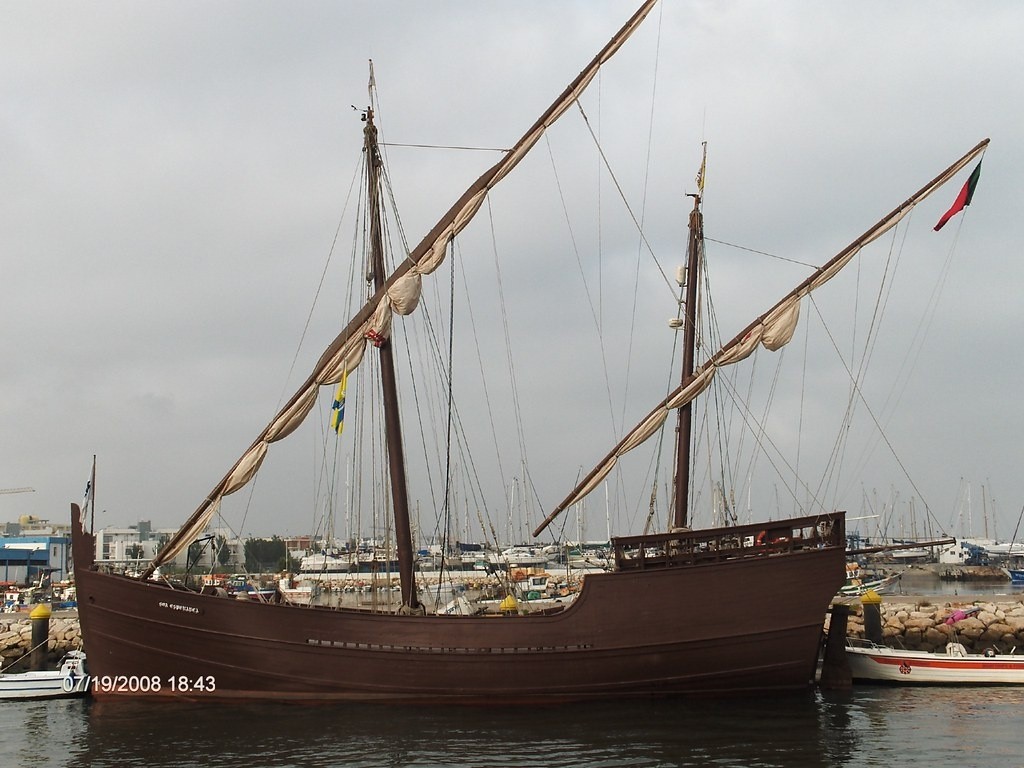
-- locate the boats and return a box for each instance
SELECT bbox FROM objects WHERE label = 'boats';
[844,636,1024,687]
[1,470,1022,616]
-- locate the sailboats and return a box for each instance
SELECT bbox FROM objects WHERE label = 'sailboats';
[69,1,995,708]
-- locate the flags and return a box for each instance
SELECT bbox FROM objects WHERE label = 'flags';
[330,363,347,434]
[934,163,981,233]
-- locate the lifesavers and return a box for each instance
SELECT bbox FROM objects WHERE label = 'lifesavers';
[756,531,780,552]
